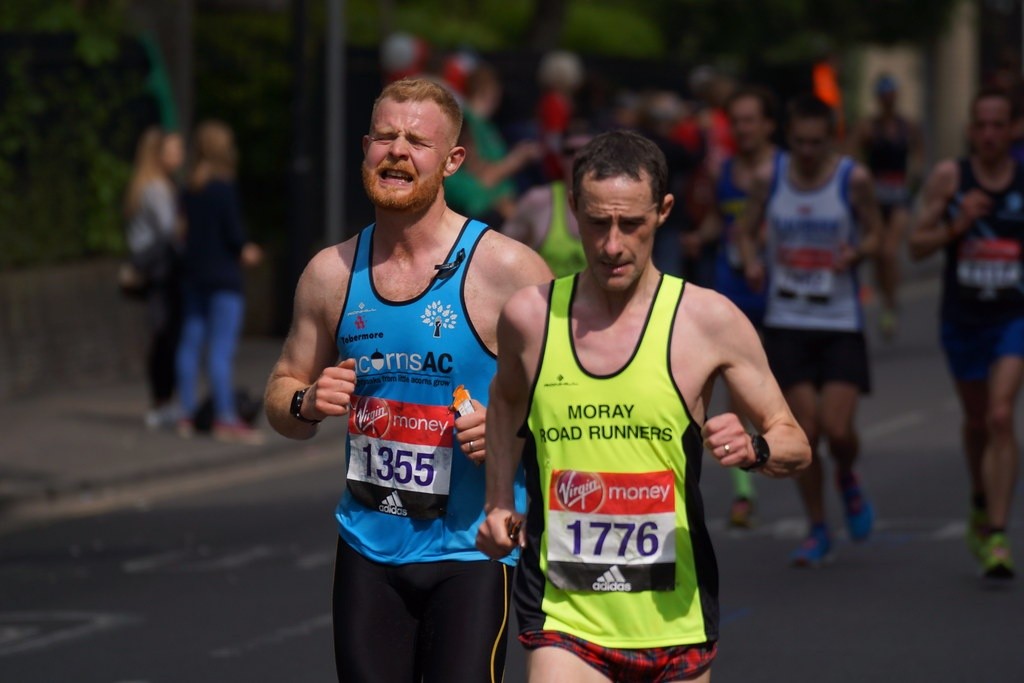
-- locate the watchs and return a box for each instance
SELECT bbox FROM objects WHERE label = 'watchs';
[740,434,771,471]
[290,387,321,423]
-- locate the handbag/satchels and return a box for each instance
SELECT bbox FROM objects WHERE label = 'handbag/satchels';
[120,242,171,297]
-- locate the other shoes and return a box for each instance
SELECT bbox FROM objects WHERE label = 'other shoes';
[178,417,200,437]
[213,423,266,446]
[146,406,186,427]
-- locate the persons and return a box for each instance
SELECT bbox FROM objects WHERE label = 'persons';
[119,123,268,440]
[385,34,924,566]
[476,129,812,683]
[910,77,1024,579]
[263,77,556,683]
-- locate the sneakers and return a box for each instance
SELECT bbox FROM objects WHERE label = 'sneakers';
[789,524,830,567]
[966,490,1015,579]
[833,464,875,540]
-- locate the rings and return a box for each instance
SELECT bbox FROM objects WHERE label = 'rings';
[470,441,474,452]
[724,444,730,453]
